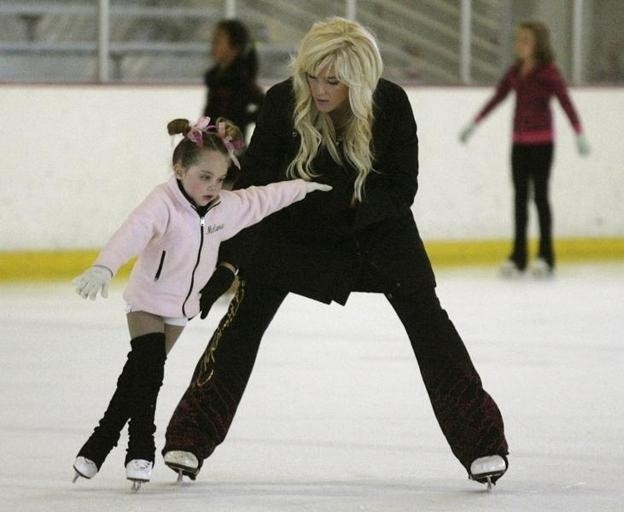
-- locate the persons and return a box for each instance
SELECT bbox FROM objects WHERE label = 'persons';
[69,119,332,493]
[459,18,593,273]
[159,15,505,491]
[198,15,265,164]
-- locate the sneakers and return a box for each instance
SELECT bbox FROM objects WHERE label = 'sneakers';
[164,451,199,470]
[470,455,506,476]
[73,457,97,478]
[126,459,152,480]
[532,260,553,277]
[499,261,528,277]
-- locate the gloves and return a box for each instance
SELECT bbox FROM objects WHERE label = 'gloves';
[199,266,235,318]
[73,266,112,300]
[460,122,476,141]
[305,181,332,193]
[315,174,361,217]
[577,136,591,156]
